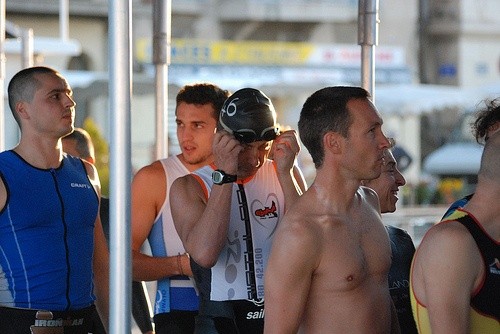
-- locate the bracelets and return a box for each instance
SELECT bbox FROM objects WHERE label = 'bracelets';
[176,253,185,276]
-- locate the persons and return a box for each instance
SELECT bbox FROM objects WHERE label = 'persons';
[169,88,307,334]
[410,96,499,334]
[132,83,244,334]
[263,85,394,333]
[60,128,154,334]
[362,136,421,333]
[0,67,109,333]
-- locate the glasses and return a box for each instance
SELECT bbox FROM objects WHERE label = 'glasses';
[220,109,280,144]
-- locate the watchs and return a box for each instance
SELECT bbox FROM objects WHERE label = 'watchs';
[211,169,237,185]
